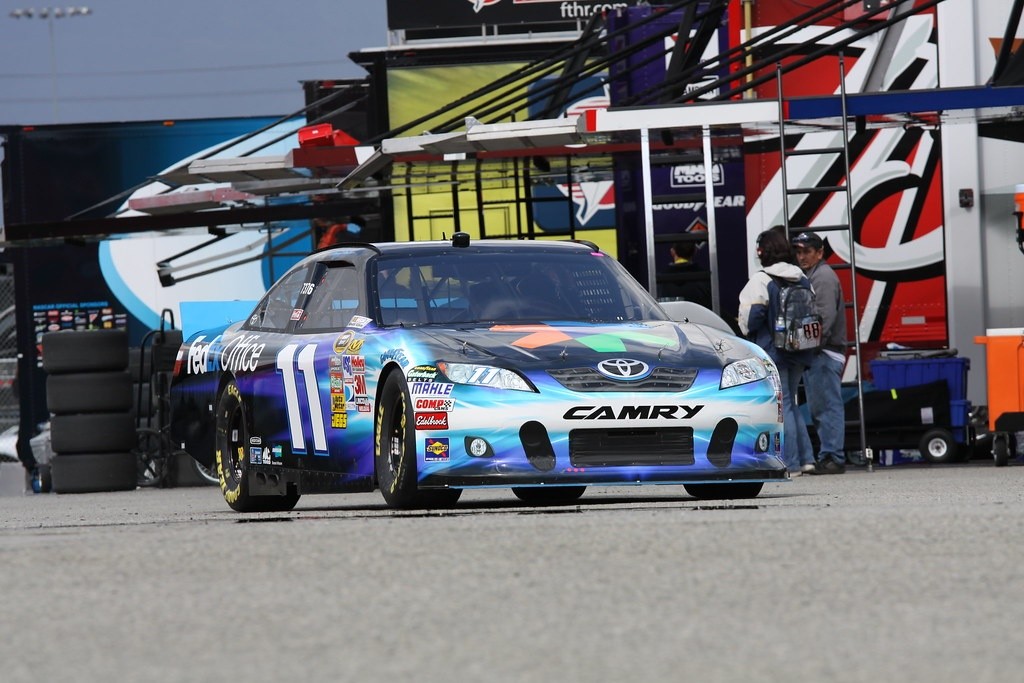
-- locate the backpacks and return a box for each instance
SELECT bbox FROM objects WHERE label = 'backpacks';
[762,269,823,361]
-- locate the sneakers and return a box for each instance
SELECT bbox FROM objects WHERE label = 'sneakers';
[810,456,845,475]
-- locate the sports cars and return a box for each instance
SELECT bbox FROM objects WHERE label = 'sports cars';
[165,235,792,510]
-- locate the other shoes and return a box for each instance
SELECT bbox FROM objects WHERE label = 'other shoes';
[784,470,803,477]
[801,464,815,472]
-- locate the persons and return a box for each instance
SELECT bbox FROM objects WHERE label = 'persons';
[792,231,847,474]
[739,225,816,477]
[663,229,712,310]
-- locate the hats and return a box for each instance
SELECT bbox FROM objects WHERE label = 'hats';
[793,231,824,248]
